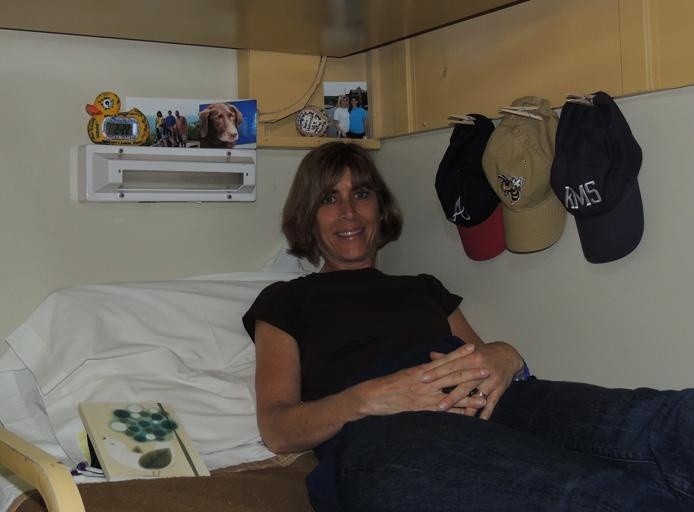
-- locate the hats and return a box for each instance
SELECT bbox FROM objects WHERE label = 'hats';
[434,114,506,261]
[550,91,644,264]
[481,96,567,253]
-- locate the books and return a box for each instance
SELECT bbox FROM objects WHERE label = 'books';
[77,399,211,482]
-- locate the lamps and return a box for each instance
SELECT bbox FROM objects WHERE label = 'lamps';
[77,140,261,206]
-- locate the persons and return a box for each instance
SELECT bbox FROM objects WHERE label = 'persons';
[334,94,349,140]
[347,96,367,140]
[242,142,693,512]
[154,108,188,147]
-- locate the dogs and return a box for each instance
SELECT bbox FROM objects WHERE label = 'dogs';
[197,103,243,149]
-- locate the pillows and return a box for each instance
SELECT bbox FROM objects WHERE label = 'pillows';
[0,275,297,471]
